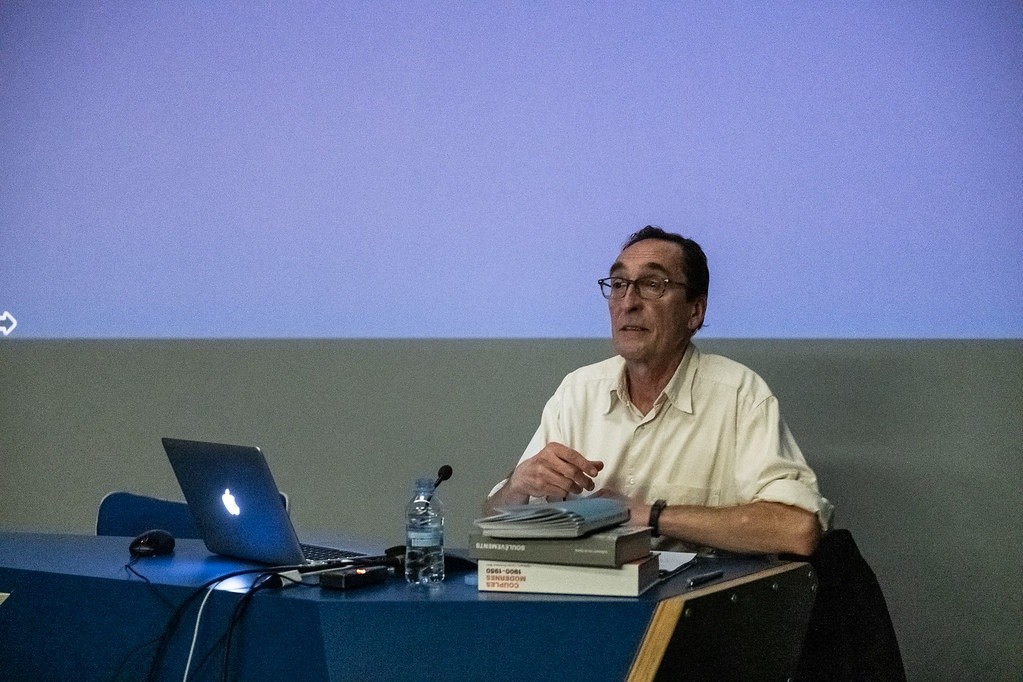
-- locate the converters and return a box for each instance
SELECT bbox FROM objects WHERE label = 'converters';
[319,565,387,590]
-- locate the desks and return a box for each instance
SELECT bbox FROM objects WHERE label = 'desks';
[1,524,906,681]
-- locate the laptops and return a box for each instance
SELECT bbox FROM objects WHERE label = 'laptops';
[161,436,377,571]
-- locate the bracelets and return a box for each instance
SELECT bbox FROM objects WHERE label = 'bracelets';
[649,496,666,539]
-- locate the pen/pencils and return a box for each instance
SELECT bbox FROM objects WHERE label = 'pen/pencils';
[686,569,726,587]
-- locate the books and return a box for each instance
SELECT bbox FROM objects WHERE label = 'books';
[479,554,665,598]
[473,498,632,538]
[466,525,654,567]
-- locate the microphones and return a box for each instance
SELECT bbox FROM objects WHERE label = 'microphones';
[420,464,453,564]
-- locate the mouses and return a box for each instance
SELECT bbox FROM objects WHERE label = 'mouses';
[128,530,175,558]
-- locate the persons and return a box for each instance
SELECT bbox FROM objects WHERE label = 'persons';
[477,225,837,556]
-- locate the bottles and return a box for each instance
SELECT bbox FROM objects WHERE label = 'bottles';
[402,477,446,586]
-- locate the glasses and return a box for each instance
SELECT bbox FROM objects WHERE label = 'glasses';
[599,276,688,300]
